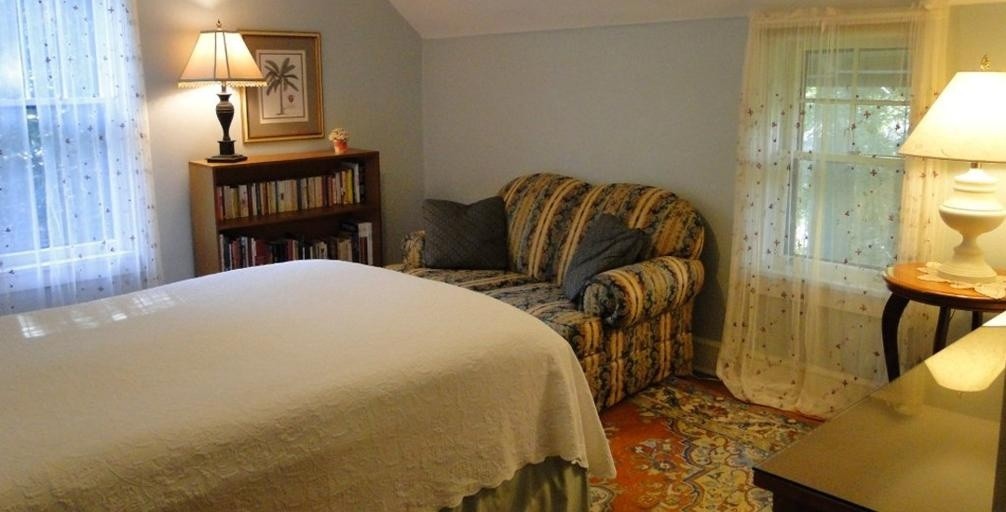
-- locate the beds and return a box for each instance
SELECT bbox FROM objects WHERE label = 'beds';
[0,256,591,511]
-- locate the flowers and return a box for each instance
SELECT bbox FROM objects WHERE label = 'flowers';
[328,126,351,143]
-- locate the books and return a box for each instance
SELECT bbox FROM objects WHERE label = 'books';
[215,160,377,274]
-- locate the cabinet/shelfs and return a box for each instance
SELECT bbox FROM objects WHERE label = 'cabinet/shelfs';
[188,148,383,278]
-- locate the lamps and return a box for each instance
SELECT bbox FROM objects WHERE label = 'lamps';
[897,53,1006,286]
[176,18,267,163]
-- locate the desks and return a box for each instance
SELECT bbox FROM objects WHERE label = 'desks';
[878,265,1006,383]
[751,310,1005,512]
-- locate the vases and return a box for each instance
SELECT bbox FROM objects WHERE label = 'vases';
[334,140,347,153]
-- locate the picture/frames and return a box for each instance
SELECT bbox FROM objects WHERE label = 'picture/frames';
[237,30,325,144]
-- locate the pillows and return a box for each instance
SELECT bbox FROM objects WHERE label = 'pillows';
[561,212,646,309]
[419,195,509,270]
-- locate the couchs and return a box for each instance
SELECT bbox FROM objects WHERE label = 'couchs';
[380,172,706,415]
